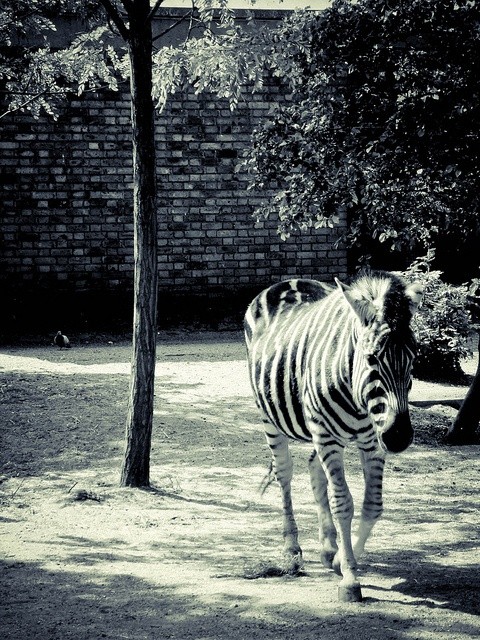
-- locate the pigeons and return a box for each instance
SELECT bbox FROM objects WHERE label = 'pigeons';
[51,330,76,352]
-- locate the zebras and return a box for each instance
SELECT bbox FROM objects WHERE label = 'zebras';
[243,272,419,601]
